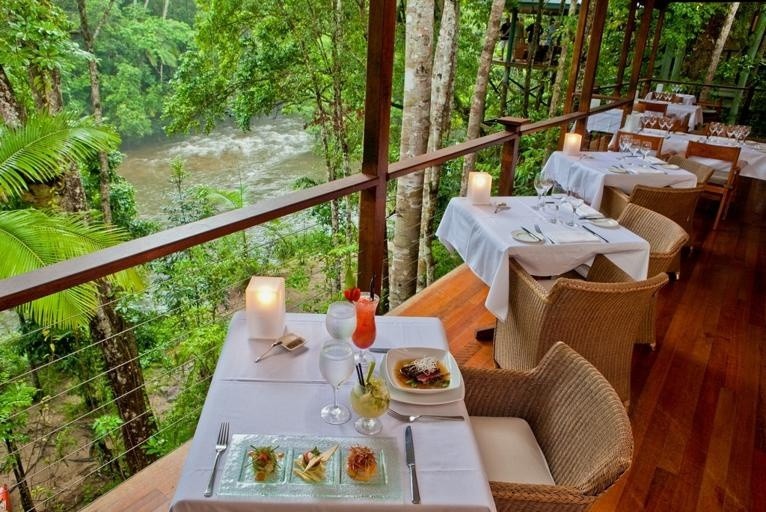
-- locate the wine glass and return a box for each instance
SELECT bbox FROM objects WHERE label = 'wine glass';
[355,292,380,369]
[350,376,390,434]
[533,172,619,244]
[609,89,750,179]
[318,339,354,425]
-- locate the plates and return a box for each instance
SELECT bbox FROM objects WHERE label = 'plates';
[512,228,546,244]
[386,347,461,393]
[380,355,466,406]
[217,433,403,500]
[279,333,306,351]
[494,201,511,209]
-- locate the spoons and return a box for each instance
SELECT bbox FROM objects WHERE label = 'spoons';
[495,202,506,213]
[254,341,283,363]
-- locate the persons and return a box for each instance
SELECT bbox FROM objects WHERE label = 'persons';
[545,18,557,48]
[498,17,511,49]
[502,17,526,62]
[523,20,543,61]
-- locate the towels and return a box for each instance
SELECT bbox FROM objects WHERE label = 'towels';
[222,320,332,382]
[544,227,591,244]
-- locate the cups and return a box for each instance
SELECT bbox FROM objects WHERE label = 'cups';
[326,300,358,340]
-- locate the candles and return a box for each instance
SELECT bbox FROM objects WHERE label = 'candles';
[563,133,582,156]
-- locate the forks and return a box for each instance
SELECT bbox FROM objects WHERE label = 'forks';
[205,421,229,497]
[386,406,465,423]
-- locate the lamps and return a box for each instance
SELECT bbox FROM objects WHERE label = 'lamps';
[468,171,491,204]
[247,272,289,340]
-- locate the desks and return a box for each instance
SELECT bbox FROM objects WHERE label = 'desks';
[177,308,497,512]
[441,194,649,337]
[543,151,698,213]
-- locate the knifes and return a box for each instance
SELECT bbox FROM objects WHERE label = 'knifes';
[369,348,389,354]
[404,426,421,503]
[521,226,542,241]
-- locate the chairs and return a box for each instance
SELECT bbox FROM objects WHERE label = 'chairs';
[622,123,766,230]
[494,253,670,400]
[585,91,704,153]
[458,345,629,512]
[603,203,689,351]
[600,184,703,280]
